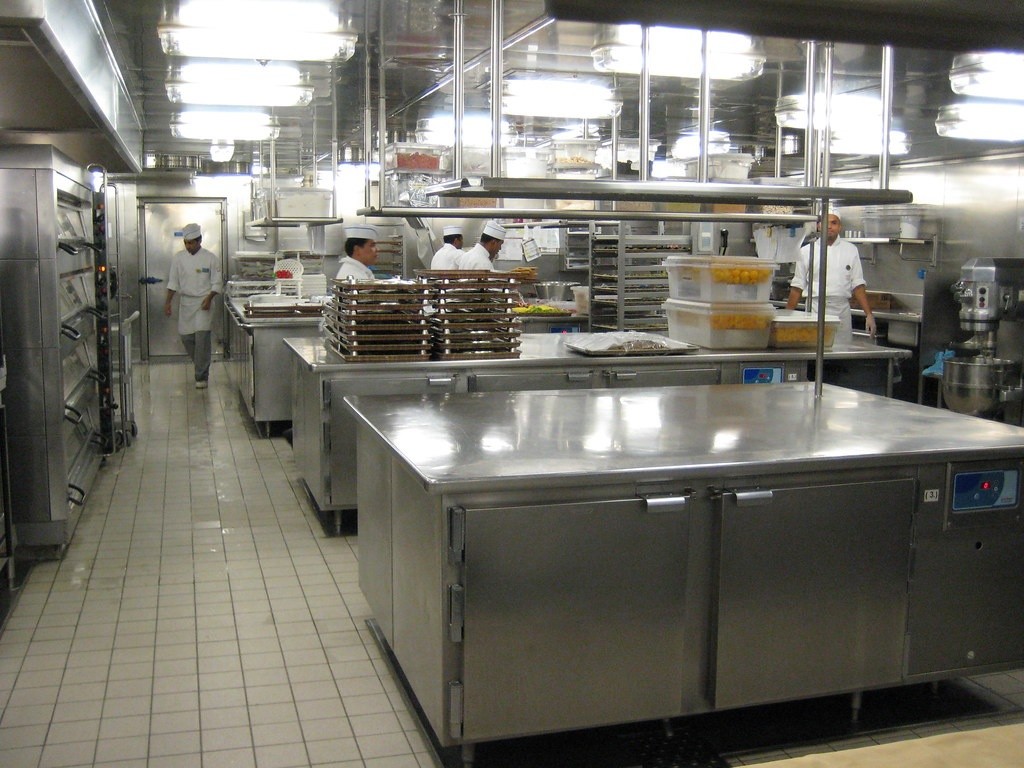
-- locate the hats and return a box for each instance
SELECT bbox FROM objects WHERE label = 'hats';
[819,205,842,222]
[443,226,463,236]
[345,223,379,239]
[183,223,201,240]
[483,220,506,241]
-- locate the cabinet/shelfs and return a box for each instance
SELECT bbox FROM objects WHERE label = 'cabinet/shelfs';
[587,221,691,338]
[558,223,590,273]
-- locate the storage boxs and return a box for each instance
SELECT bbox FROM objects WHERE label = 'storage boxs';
[661,256,840,350]
[251,187,333,219]
[684,154,756,180]
[381,138,660,183]
[859,205,942,240]
[437,195,794,215]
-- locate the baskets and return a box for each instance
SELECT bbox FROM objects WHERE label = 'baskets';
[273,259,304,278]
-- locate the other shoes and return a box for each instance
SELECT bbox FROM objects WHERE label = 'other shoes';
[196,380,209,389]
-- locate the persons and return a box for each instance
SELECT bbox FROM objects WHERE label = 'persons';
[458,220,506,312]
[163,222,223,388]
[786,209,876,339]
[430,226,464,312]
[333,224,380,289]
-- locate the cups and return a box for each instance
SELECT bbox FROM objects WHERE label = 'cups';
[569,286,589,315]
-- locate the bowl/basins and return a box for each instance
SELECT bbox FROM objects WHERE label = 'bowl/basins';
[533,281,580,302]
